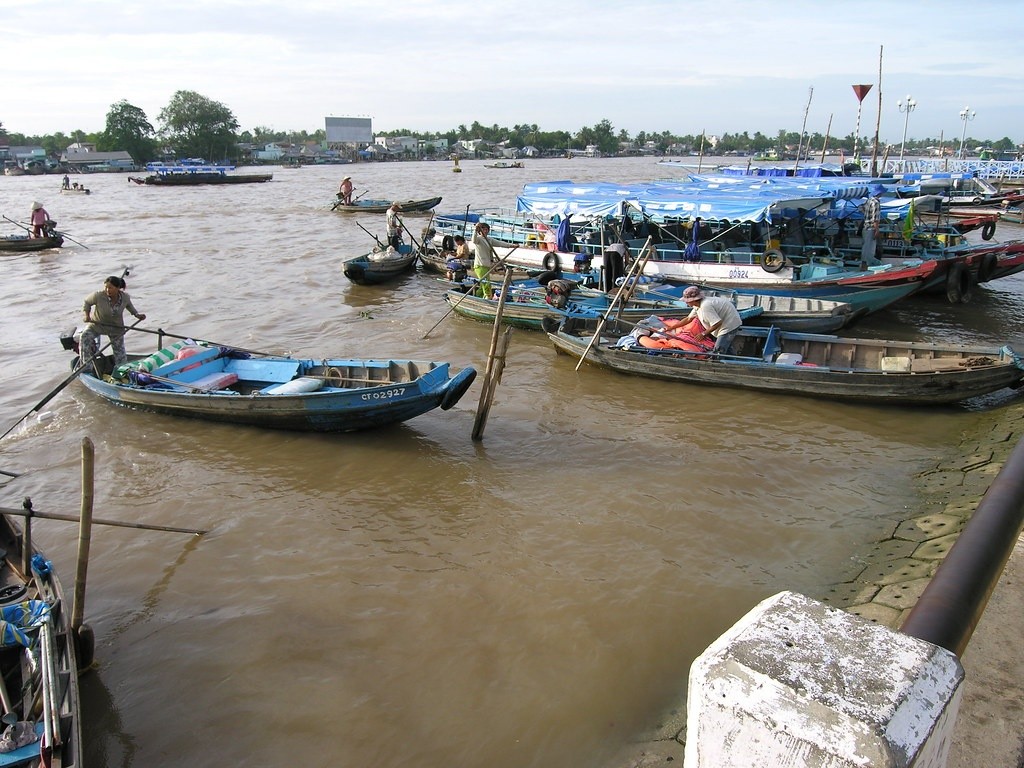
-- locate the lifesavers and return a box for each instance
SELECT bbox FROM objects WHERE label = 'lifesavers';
[981,220,996,241]
[442,235,453,250]
[760,249,784,272]
[542,251,559,271]
[944,262,975,305]
[976,253,999,283]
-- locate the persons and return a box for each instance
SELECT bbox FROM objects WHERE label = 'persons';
[30,201,49,238]
[339,176,352,205]
[603,242,630,291]
[386,201,402,251]
[451,235,469,260]
[860,184,889,270]
[472,223,520,299]
[786,208,809,256]
[63,175,69,189]
[663,286,742,360]
[79,276,146,373]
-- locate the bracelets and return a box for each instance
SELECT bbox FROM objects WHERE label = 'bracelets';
[702,332,706,336]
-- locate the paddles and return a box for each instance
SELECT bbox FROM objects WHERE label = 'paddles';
[1,507,206,535]
[356,222,385,247]
[392,210,421,248]
[476,222,516,286]
[352,190,368,203]
[86,317,278,358]
[20,220,74,237]
[1,314,148,441]
[624,244,657,302]
[330,188,357,211]
[576,235,650,370]
[421,244,521,339]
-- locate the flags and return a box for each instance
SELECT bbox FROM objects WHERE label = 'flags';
[903,200,914,242]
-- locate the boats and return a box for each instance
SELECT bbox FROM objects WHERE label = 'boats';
[59,318,478,432]
[0,232,64,252]
[60,187,90,196]
[752,142,783,161]
[484,160,526,168]
[541,311,1024,407]
[723,149,750,157]
[0,432,85,768]
[783,145,815,161]
[507,182,1024,307]
[127,170,274,185]
[442,284,854,335]
[341,243,417,286]
[584,139,601,158]
[334,196,442,214]
[452,154,462,172]
[417,245,553,282]
[430,212,939,317]
[891,172,1024,212]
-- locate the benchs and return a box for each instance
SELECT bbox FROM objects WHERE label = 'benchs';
[179,373,237,391]
[268,376,325,396]
[776,353,803,365]
[0,721,44,768]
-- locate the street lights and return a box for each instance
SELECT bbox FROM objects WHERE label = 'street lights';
[958,105,977,160]
[897,94,917,160]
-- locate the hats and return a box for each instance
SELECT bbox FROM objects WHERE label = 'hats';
[869,184,888,196]
[680,286,705,302]
[31,201,42,210]
[341,176,352,181]
[392,200,402,209]
